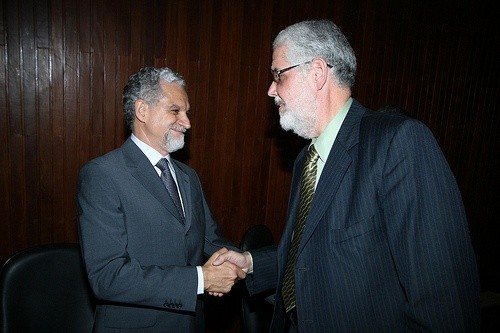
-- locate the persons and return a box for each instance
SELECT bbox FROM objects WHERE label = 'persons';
[76,66,245,333]
[208,20,482,333]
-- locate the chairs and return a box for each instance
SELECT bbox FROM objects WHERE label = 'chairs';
[239,223,276,333]
[0,243,96,333]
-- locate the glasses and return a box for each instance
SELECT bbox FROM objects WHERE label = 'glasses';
[272,60,333,84]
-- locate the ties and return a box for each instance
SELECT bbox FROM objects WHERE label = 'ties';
[156,158,186,225]
[281,144,319,313]
